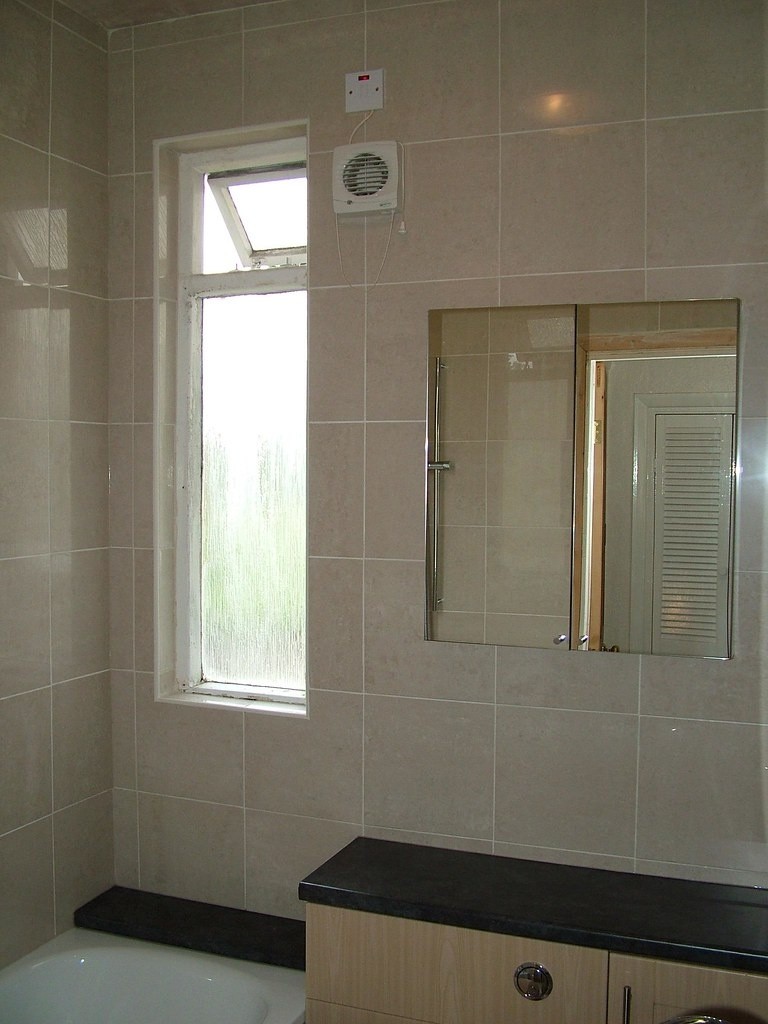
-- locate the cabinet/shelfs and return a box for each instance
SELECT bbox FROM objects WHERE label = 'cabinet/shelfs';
[296,838,768,1024]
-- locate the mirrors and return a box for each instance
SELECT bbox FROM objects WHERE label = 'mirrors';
[424,298,739,661]
